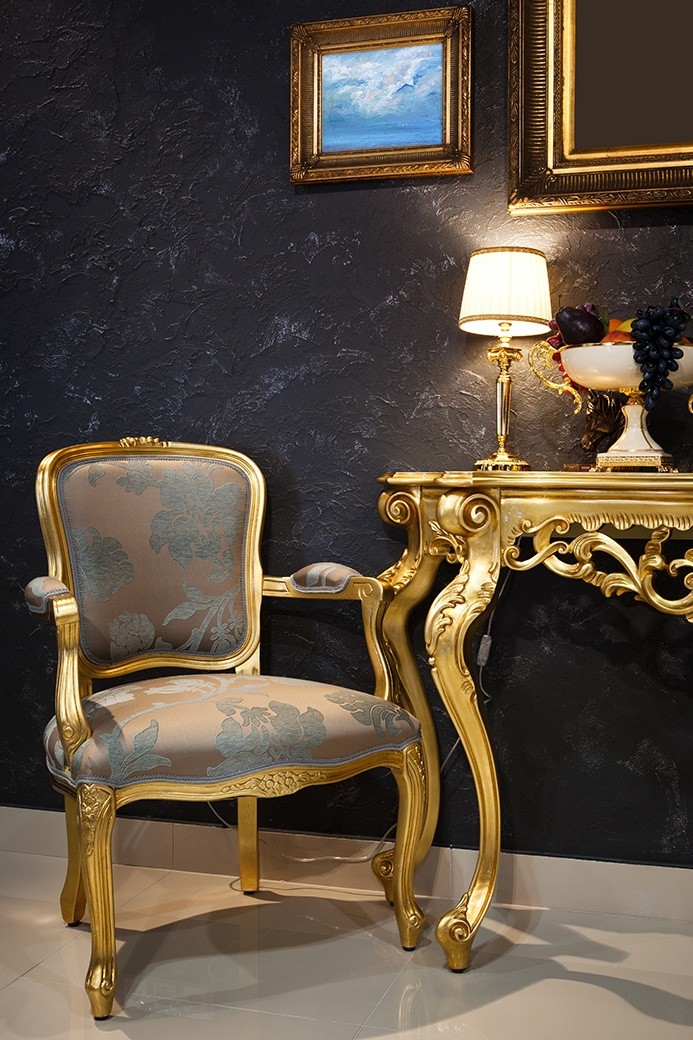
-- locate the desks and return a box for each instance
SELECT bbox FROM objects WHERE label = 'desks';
[371,471,693,973]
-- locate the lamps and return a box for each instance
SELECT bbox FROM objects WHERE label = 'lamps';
[458,245,553,470]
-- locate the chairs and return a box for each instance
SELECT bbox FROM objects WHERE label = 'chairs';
[24,436,431,1018]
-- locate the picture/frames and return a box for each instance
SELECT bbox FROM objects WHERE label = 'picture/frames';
[507,0,693,216]
[289,5,473,186]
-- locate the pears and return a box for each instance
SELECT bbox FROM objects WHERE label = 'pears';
[554,295,605,343]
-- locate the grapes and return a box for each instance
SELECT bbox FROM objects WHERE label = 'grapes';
[547,302,595,395]
[630,297,687,411]
